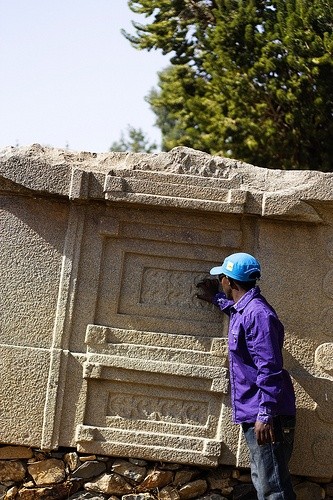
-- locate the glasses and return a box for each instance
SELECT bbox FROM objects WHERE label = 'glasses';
[218,274,226,282]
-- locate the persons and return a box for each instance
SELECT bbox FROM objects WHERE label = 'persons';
[194,253,296,500]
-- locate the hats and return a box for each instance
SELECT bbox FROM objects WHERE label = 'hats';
[210,253,262,281]
